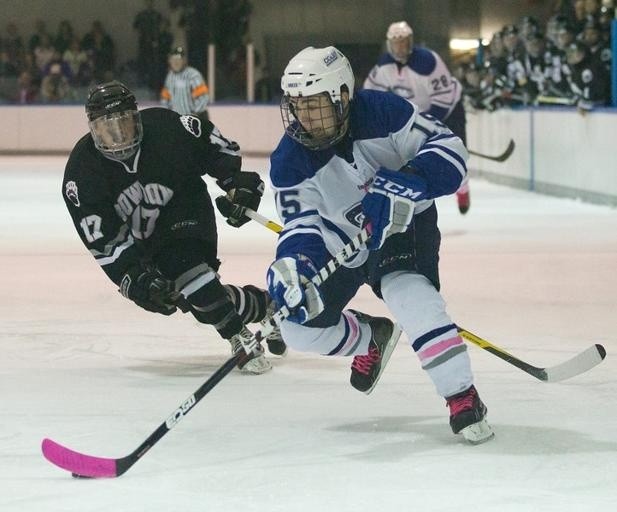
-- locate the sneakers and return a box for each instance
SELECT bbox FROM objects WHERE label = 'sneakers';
[227,291,287,370]
[350,312,393,391]
[442,384,487,433]
[458,191,470,213]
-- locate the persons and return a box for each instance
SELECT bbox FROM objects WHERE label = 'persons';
[266,45,488,435]
[61,79,287,370]
[451,0,617,116]
[0,0,284,121]
[361,20,470,216]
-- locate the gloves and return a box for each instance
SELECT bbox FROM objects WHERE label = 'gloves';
[361,166,427,251]
[266,254,326,325]
[215,172,265,227]
[120,264,177,316]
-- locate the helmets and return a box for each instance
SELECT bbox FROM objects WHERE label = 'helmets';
[387,21,413,40]
[86,80,144,161]
[278,44,356,153]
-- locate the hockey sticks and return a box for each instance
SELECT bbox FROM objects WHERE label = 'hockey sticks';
[42,224,372,478]
[467,140,515,162]
[225,195,606,382]
